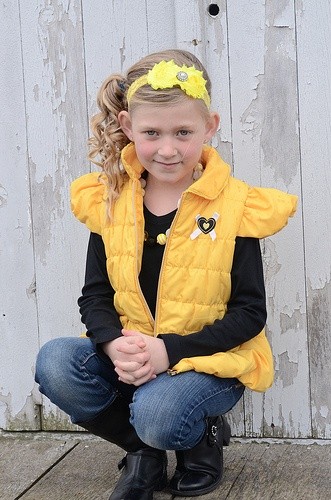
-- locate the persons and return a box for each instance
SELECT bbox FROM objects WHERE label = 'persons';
[32,48,297,498]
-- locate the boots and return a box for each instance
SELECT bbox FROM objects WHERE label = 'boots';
[168,416,231,497]
[71,391,168,500]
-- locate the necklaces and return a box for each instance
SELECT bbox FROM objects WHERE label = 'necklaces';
[137,161,205,247]
